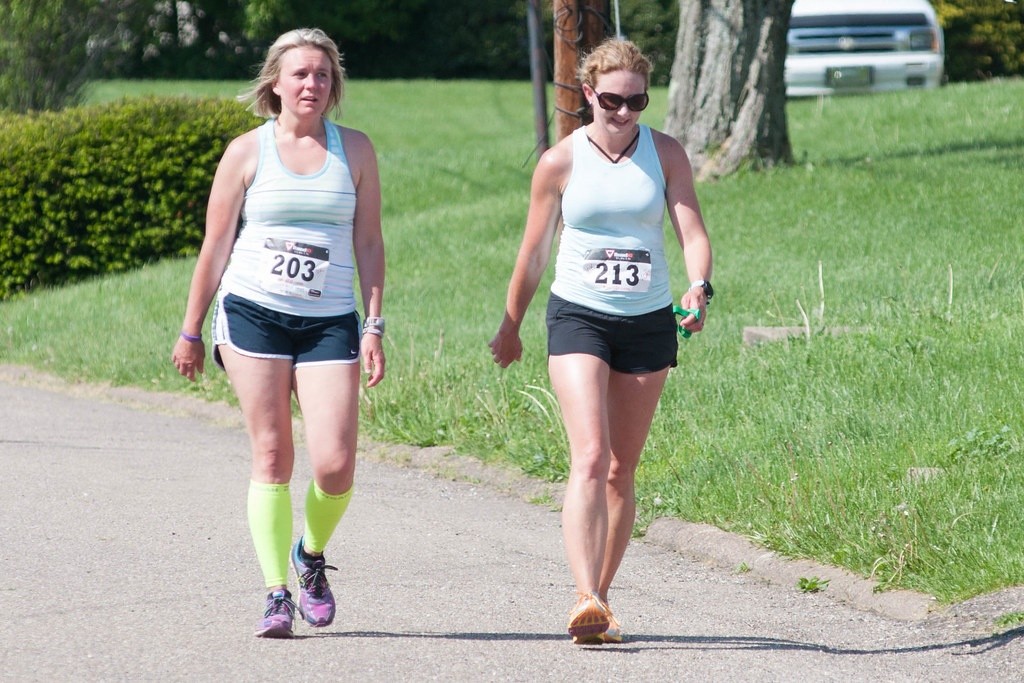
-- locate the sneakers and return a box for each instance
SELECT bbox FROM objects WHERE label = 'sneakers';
[289,536,338,627]
[569,589,610,646]
[255,590,305,638]
[601,602,622,643]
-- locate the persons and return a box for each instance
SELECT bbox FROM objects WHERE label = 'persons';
[486,40,712,644]
[172,31,387,639]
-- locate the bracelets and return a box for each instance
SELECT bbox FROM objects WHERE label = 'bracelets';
[181,331,202,342]
[362,328,382,337]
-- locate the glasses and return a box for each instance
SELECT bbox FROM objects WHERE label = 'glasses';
[587,85,650,112]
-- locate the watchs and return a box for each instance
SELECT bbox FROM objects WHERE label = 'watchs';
[362,317,385,332]
[689,280,714,299]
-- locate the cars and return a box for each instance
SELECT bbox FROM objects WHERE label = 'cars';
[782,0,945,96]
